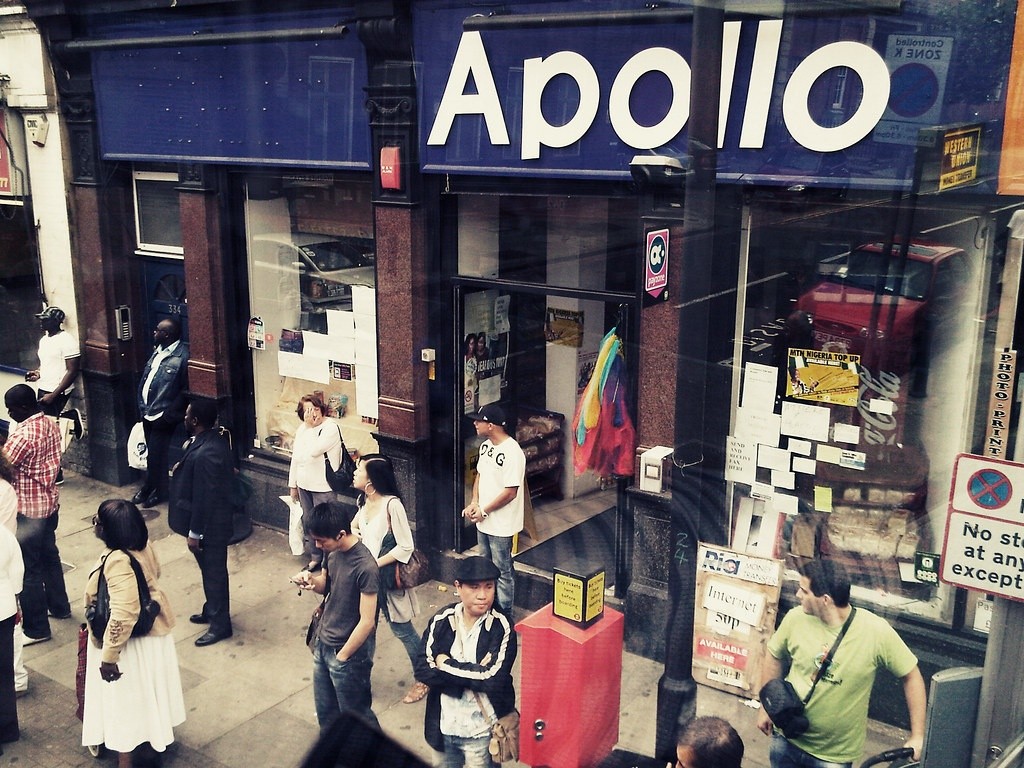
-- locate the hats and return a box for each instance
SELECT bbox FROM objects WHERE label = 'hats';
[455,556,501,583]
[468,404,506,427]
[34,306,65,324]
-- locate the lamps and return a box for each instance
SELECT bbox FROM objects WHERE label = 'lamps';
[629,155,685,200]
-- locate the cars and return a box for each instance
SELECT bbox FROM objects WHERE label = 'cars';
[252,234,372,287]
[794,241,975,377]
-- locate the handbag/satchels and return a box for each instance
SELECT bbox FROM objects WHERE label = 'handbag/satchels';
[318,422,357,493]
[40,402,75,453]
[759,678,810,738]
[125,421,149,471]
[385,497,434,589]
[487,710,520,764]
[279,495,305,556]
[306,553,329,654]
[84,548,161,641]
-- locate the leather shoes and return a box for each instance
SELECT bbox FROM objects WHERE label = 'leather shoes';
[190,613,213,624]
[194,632,231,646]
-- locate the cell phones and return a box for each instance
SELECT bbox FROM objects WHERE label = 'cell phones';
[290,576,310,586]
[25,373,36,380]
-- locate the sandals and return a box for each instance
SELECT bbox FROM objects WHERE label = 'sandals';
[403,681,430,704]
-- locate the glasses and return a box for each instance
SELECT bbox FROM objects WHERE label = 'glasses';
[91,515,105,532]
[152,326,170,336]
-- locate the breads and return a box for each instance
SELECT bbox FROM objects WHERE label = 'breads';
[516,414,560,473]
[828,486,922,559]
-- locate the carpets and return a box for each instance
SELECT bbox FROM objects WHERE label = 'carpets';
[512,504,617,589]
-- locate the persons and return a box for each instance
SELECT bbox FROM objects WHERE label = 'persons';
[287,395,431,733]
[752,559,927,768]
[0,384,72,756]
[132,317,188,509]
[168,399,236,647]
[461,403,526,617]
[666,716,743,768]
[24,304,84,485]
[416,556,518,768]
[466,331,491,378]
[787,365,818,398]
[80,499,185,768]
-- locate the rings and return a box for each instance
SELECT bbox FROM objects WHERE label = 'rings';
[110,673,113,678]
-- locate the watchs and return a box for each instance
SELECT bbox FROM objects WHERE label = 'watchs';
[481,509,489,520]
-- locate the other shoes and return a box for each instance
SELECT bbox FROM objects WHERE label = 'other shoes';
[21,627,52,647]
[61,408,85,440]
[47,607,72,619]
[132,480,169,508]
[301,561,321,572]
[54,468,64,486]
